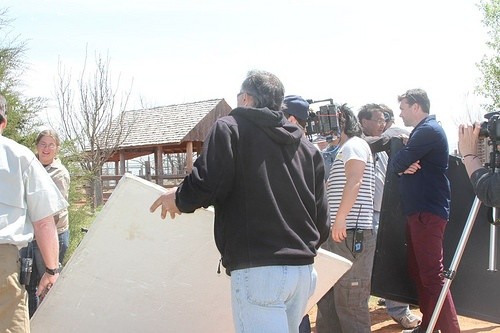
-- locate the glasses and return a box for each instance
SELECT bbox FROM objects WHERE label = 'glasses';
[37,142,57,148]
[403,90,418,103]
[367,118,387,124]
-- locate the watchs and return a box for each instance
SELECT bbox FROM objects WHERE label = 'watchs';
[46,263,64,274]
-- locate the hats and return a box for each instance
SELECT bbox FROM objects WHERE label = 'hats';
[0,94,6,119]
[282,95,310,120]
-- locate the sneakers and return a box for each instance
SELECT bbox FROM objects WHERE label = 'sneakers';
[391,312,422,328]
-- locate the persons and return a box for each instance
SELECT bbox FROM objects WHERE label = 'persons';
[357,103,422,328]
[317,106,375,333]
[459,119,500,207]
[0,94,63,333]
[20,129,71,318]
[281,95,309,137]
[150,71,331,333]
[387,88,461,333]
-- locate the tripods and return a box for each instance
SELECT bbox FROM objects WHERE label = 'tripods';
[425,145,500,333]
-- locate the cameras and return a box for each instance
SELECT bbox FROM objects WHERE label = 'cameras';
[472,111,500,145]
